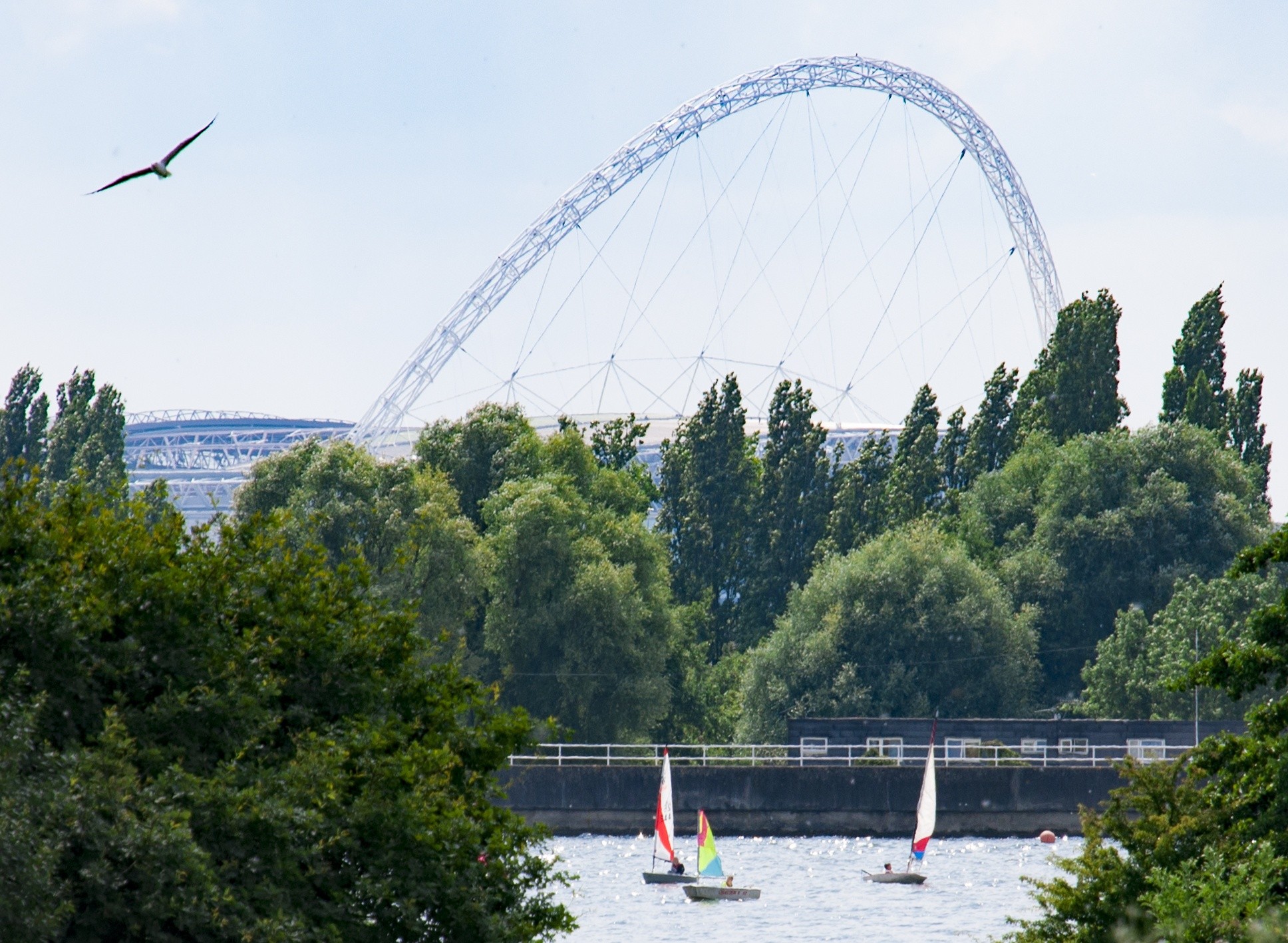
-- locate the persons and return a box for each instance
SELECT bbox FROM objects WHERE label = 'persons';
[667,857,684,875]
[884,862,894,874]
[722,876,733,887]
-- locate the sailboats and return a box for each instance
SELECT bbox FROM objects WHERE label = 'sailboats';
[682,803,762,903]
[642,742,700,885]
[859,705,941,885]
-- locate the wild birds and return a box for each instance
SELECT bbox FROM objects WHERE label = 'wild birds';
[84,112,220,196]
